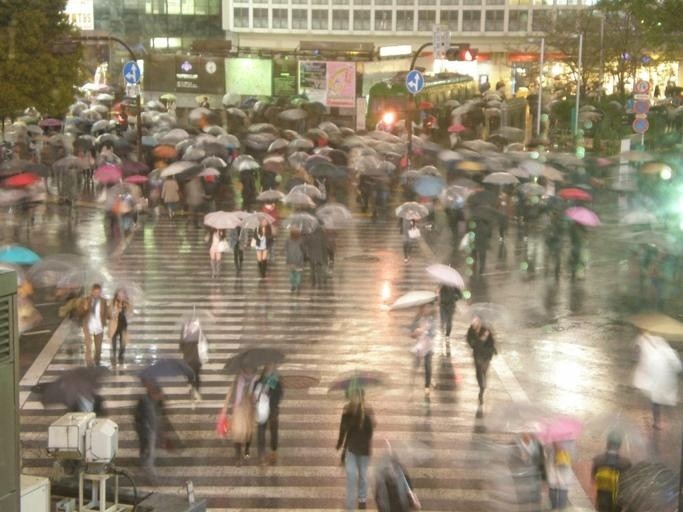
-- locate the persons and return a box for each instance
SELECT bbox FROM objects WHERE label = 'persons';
[0,235,130,370]
[177,311,207,399]
[335,388,376,510]
[434,285,461,334]
[631,312,682,431]
[72,381,107,416]
[467,314,496,403]
[411,301,449,392]
[218,361,286,462]
[393,198,591,281]
[376,442,416,512]
[204,208,351,293]
[504,402,575,511]
[138,379,165,477]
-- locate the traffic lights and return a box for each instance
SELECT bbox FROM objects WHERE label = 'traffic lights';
[445,47,479,61]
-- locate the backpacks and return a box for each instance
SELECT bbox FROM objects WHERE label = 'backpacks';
[594,452,622,511]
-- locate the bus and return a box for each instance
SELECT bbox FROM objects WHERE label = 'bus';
[364,71,475,130]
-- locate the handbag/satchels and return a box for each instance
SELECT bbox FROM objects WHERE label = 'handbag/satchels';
[407,226,420,239]
[198,338,209,364]
[217,239,231,253]
[217,413,231,439]
